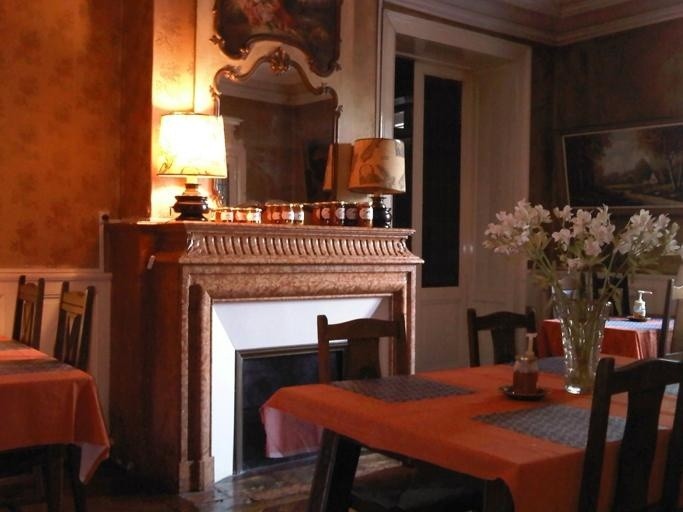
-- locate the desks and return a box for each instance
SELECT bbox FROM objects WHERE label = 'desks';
[0,333,113,512]
[256,353,682,512]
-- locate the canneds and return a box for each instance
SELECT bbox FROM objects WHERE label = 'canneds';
[211,201,373,226]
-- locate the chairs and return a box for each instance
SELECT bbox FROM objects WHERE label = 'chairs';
[0,280,96,512]
[463,309,546,360]
[0,274,46,498]
[316,314,412,382]
[573,355,682,510]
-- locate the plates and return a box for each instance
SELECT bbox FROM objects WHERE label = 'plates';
[499,385,547,403]
[627,316,651,322]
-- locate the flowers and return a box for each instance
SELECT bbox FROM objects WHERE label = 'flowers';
[480,195,683,381]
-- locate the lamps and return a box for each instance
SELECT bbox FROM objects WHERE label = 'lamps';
[347,137,406,228]
[155,110,229,222]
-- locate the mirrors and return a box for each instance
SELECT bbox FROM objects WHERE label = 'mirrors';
[209,45,343,212]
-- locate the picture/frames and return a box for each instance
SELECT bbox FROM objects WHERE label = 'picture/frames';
[562,123,683,209]
